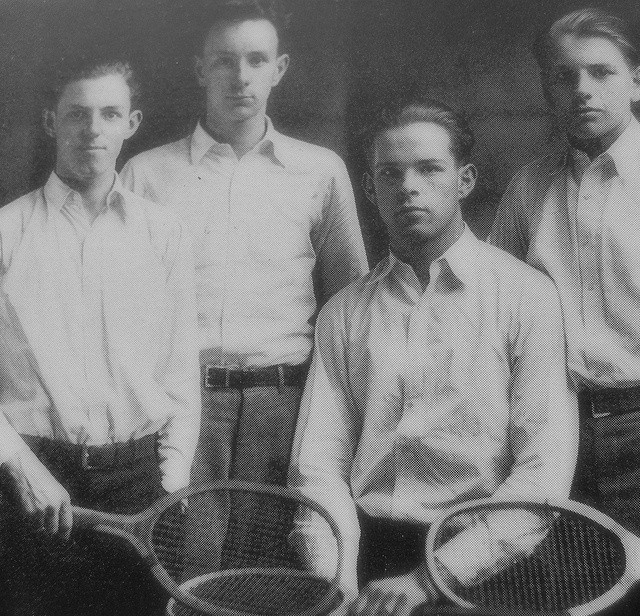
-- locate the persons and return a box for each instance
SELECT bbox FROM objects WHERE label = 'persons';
[116,0,371,583]
[485,8,639,532]
[0,43,204,614]
[286,96,581,616]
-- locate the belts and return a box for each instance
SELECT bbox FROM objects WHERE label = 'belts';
[578,386,640,415]
[207,365,312,389]
[19,431,160,471]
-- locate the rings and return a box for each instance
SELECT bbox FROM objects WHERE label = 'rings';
[28,522,45,535]
